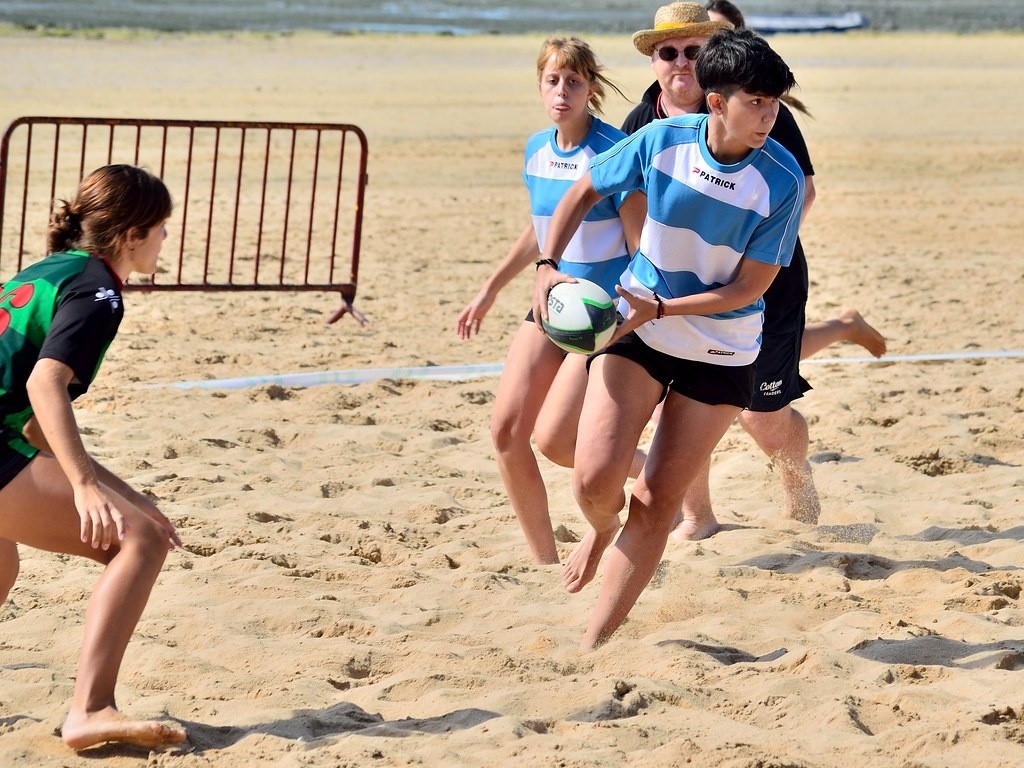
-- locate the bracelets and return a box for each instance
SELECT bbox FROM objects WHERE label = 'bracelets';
[651,292,662,320]
[660,304,665,318]
[536,258,558,271]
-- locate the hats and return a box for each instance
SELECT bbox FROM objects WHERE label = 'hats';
[632,2,735,58]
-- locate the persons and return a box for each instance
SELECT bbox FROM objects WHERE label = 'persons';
[0,165,189,750]
[454,0,886,653]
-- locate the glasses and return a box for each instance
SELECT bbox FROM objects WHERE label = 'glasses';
[653,44,701,61]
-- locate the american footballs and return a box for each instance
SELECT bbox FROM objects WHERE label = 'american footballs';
[540,277,617,356]
